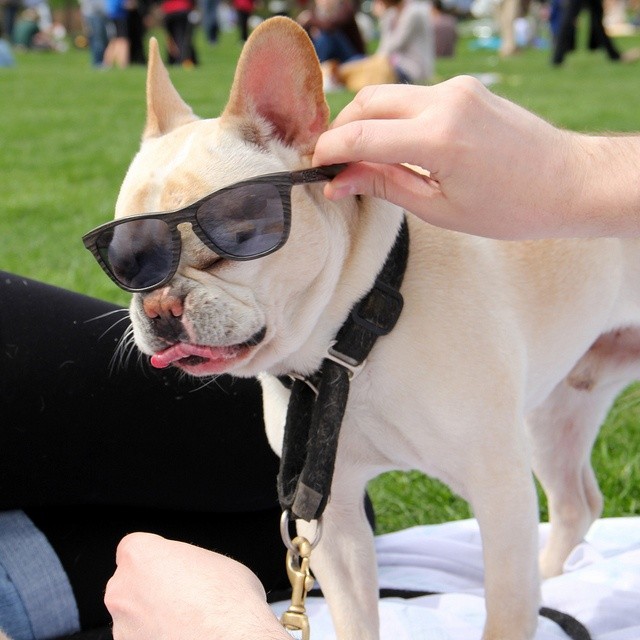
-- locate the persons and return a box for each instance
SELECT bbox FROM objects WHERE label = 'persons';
[0,1,290,67]
[298,0,640,93]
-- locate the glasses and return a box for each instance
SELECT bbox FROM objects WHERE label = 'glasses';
[82,165,365,294]
